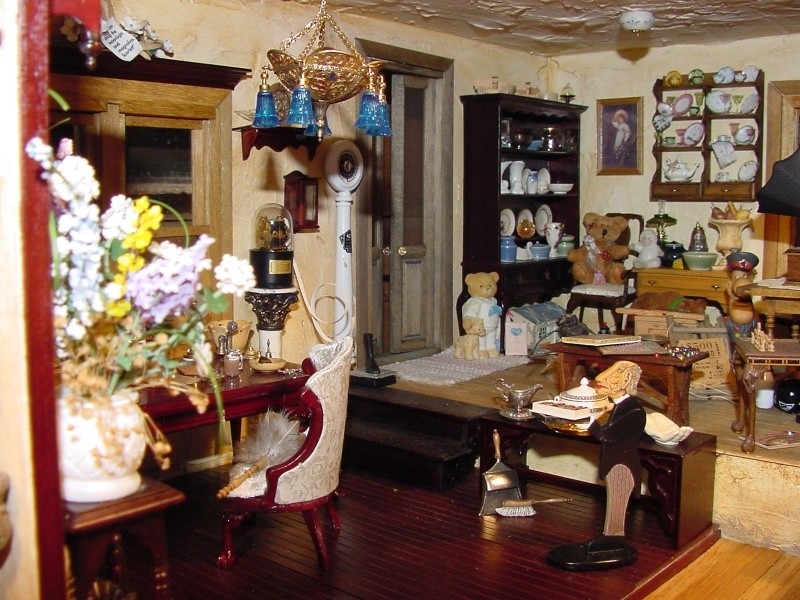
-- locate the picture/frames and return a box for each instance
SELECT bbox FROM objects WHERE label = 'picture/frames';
[596,96,644,175]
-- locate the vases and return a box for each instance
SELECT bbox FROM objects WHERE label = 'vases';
[53,383,148,503]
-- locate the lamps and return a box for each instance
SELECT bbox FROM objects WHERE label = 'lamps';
[647,200,678,245]
[251,0,393,143]
[618,11,654,37]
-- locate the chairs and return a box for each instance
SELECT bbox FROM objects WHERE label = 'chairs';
[215,336,355,572]
[566,212,645,335]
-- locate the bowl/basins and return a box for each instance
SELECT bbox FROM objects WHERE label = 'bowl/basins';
[208,319,253,355]
[250,358,286,373]
[682,251,718,271]
[547,183,573,194]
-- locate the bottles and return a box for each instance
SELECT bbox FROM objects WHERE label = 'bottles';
[557,233,575,256]
[554,378,609,431]
[663,243,686,267]
[526,171,538,194]
[688,223,708,252]
[509,161,525,188]
[543,126,559,152]
[500,235,517,263]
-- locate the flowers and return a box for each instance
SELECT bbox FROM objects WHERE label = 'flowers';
[25,135,257,478]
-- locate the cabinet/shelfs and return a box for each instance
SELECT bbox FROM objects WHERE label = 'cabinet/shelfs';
[456,93,588,355]
[650,68,764,203]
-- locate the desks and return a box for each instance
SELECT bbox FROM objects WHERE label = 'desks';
[476,268,800,551]
[132,356,310,435]
[61,478,184,600]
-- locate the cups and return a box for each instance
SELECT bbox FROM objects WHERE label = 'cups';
[224,356,241,376]
[716,171,729,180]
[512,132,527,150]
[531,241,550,260]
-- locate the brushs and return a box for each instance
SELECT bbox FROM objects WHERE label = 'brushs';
[495,495,574,517]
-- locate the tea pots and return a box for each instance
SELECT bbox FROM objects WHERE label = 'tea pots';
[663,158,700,180]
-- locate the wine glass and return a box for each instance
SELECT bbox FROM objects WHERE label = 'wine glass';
[544,223,564,258]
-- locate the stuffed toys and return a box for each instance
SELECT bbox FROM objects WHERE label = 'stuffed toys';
[566,213,629,285]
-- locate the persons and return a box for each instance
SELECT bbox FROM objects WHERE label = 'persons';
[587,359,646,552]
[628,228,663,269]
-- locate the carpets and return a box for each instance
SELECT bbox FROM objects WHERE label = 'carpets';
[378,343,534,386]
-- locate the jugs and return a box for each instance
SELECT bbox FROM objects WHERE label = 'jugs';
[495,377,543,414]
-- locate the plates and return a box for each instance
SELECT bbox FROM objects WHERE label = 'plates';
[737,161,758,180]
[500,205,552,237]
[522,169,551,192]
[715,179,731,183]
[664,65,759,146]
[640,334,667,347]
[666,179,692,183]
